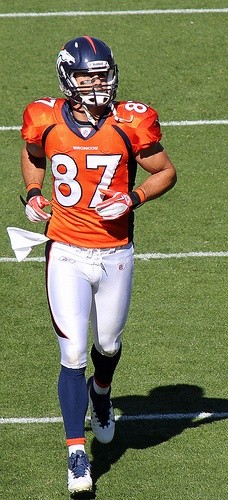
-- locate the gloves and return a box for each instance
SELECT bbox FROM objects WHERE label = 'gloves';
[20,187,53,223]
[95,188,140,219]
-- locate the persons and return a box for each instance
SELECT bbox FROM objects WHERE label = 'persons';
[20,36,177,493]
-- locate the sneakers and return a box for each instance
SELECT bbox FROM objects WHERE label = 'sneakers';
[88,375,116,444]
[67,449,93,494]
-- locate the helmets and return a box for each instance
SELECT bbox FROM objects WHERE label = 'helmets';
[56,36,120,108]
